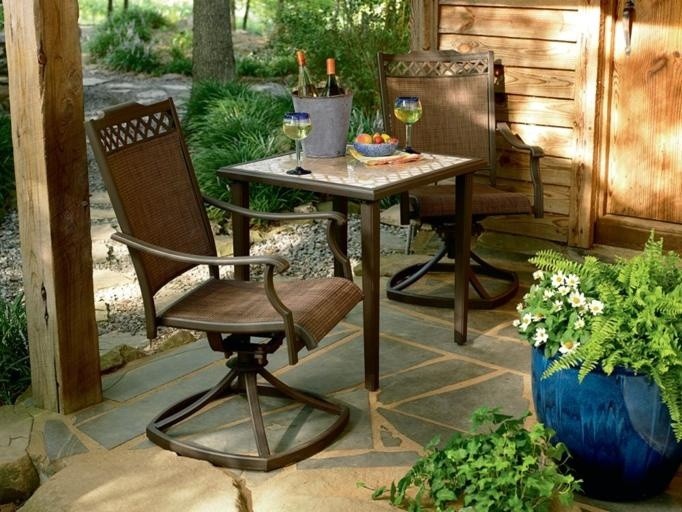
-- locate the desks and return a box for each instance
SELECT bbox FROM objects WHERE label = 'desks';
[214,144,491,396]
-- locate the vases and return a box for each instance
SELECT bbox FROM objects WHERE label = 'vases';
[529,344,675,503]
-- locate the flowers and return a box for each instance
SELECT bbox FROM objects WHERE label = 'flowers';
[513,227,682,455]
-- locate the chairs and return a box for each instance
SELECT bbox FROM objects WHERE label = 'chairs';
[373,48,547,313]
[81,95,368,474]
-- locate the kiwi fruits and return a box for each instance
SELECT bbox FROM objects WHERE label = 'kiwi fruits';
[373,132,381,140]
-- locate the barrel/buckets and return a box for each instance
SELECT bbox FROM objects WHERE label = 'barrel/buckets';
[290,88,354,158]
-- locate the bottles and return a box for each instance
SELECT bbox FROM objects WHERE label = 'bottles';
[293,49,343,98]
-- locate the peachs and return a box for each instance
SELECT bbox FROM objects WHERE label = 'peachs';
[356,133,372,144]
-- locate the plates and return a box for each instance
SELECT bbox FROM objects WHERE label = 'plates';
[345,145,418,166]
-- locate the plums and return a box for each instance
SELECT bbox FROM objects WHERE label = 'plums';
[375,136,384,143]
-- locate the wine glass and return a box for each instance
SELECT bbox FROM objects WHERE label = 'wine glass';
[393,94,423,154]
[282,112,313,176]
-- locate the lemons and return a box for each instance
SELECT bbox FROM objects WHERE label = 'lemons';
[381,132,391,141]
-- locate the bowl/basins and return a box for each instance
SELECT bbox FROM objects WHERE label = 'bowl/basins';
[353,140,400,157]
